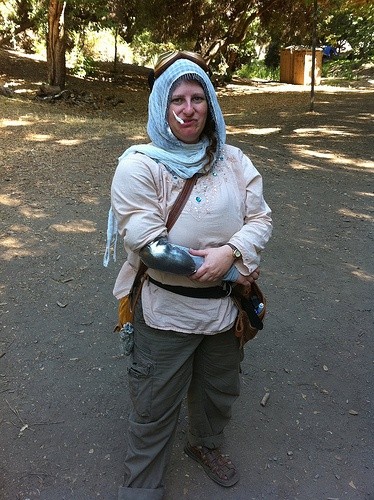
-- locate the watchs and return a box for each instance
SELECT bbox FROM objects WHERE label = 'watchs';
[226,243,241,259]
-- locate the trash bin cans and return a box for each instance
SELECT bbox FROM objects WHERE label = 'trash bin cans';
[280,45,323,86]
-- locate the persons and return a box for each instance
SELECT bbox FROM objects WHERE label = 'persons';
[102,51,274,499]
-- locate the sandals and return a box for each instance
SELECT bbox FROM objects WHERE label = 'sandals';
[184,441,239,486]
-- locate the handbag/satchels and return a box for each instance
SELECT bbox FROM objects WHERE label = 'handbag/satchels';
[119,296,133,329]
[234,283,267,346]
